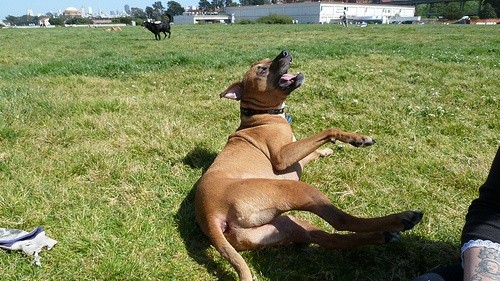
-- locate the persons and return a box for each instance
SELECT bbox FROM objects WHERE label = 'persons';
[341,7,349,27]
[414,147,500,281]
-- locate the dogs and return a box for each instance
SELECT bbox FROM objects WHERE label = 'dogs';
[141,13,171,40]
[195,50,424,281]
